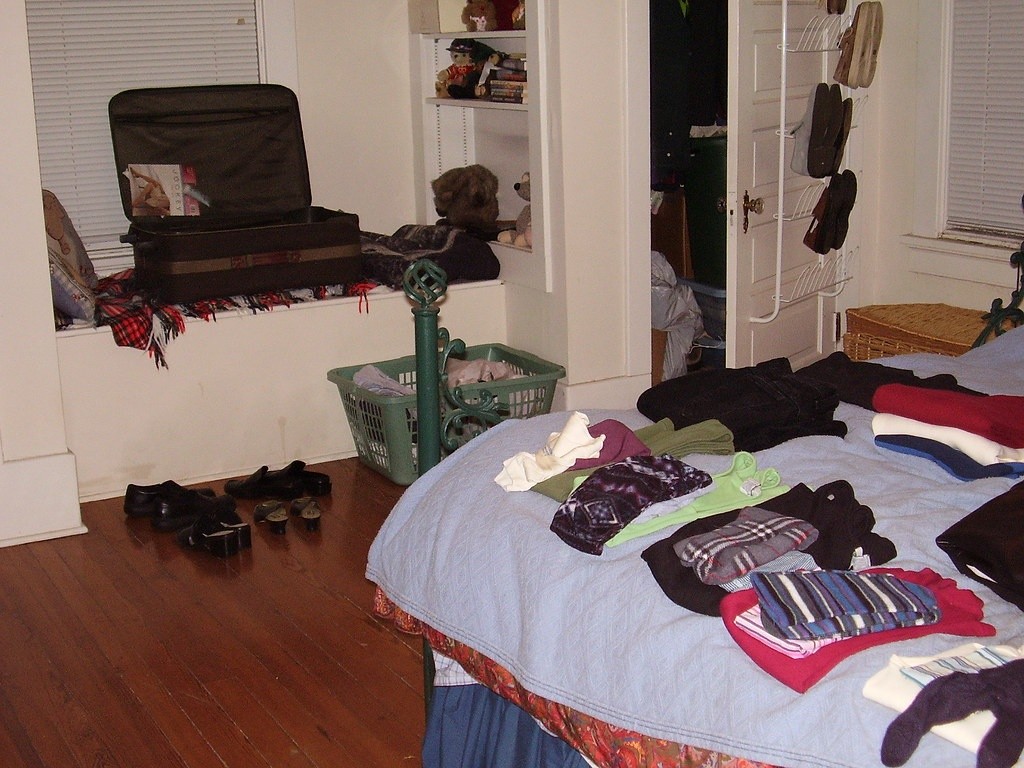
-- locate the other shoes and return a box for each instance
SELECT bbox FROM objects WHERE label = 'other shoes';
[790,3,884,257]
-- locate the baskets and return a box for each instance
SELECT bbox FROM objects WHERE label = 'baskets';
[327,342,566,486]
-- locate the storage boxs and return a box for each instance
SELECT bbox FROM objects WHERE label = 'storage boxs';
[651,279,696,390]
[326,342,567,486]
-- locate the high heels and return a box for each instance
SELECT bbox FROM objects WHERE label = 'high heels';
[215,512,252,549]
[253,500,288,534]
[177,516,240,559]
[289,497,320,531]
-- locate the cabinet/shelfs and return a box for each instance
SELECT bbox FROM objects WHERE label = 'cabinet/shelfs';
[408,0,553,295]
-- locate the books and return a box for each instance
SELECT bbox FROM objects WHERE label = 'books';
[487,53,528,105]
[128,163,200,216]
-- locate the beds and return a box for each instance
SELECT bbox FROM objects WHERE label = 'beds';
[365,323,1024,768]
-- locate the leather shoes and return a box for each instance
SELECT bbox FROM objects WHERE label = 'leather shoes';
[124,482,214,519]
[152,490,238,533]
[264,460,332,496]
[225,466,303,500]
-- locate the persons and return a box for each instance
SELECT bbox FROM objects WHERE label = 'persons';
[130,166,168,208]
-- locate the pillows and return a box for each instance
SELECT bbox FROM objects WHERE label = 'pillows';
[41,188,98,324]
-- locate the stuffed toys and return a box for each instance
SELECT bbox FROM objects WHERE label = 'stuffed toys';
[430,164,500,229]
[435,39,508,102]
[460,0,526,32]
[497,171,532,249]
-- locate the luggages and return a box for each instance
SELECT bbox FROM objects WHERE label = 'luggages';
[109,83,364,303]
